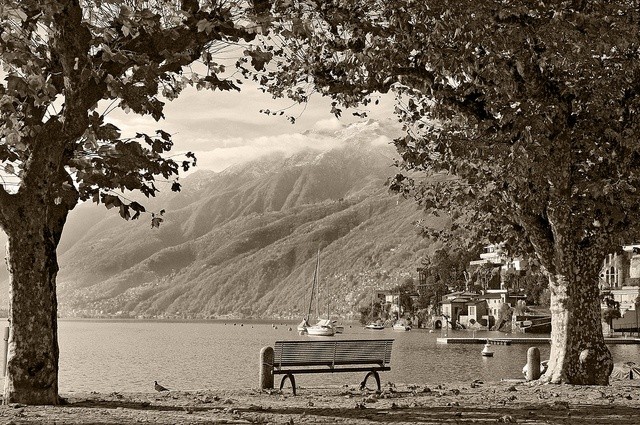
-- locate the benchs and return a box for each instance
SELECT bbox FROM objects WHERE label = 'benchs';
[270,339,395,397]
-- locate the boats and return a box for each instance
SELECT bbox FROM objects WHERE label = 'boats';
[366,322,384,329]
[393,323,411,330]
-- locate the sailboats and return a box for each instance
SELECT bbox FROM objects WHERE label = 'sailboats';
[299,242,335,336]
[326,277,345,335]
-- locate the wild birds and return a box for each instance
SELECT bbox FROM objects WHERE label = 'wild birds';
[154,381,169,392]
